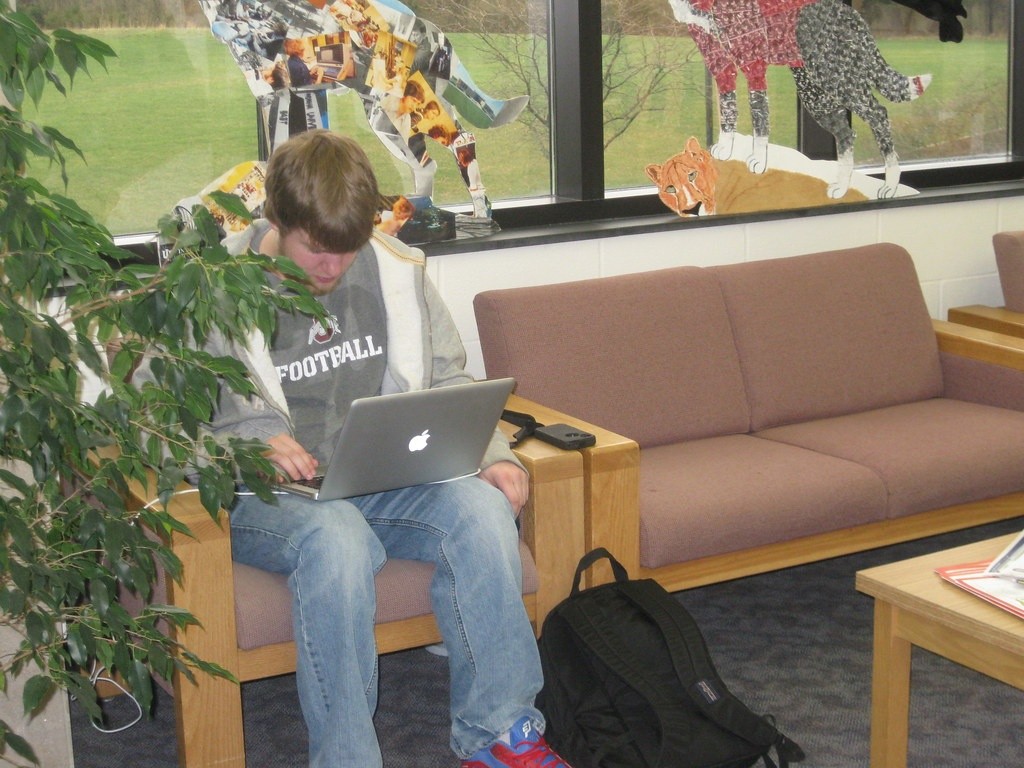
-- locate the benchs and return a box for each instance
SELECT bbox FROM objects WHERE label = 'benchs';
[474,243,1024,592]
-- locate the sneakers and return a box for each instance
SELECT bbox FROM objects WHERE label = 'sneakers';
[460,716,572,768]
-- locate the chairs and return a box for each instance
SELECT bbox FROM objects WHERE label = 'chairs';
[60,416,584,768]
[948,230,1024,339]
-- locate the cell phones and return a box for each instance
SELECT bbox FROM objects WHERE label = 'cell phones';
[530,420,597,450]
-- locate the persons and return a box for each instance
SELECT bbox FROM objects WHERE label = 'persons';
[124,129,575,768]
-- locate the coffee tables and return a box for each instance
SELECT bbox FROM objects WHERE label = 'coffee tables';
[856,532,1024,768]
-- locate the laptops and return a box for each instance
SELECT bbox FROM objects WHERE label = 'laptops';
[263,374,518,502]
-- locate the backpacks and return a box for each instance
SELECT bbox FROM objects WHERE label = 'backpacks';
[534,548,806,768]
